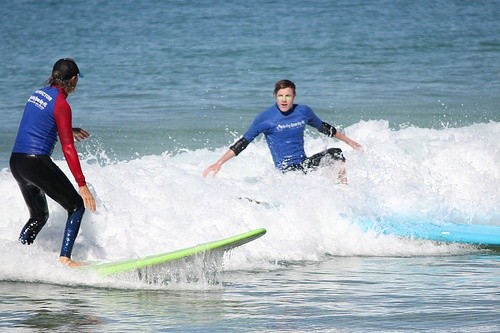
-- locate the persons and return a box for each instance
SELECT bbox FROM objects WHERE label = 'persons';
[9,57,98,267]
[203,79,364,186]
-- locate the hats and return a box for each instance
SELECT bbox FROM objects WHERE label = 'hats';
[52,57,84,80]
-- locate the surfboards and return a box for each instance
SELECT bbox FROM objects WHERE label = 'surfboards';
[238,193,499,245]
[73,227,266,275]
